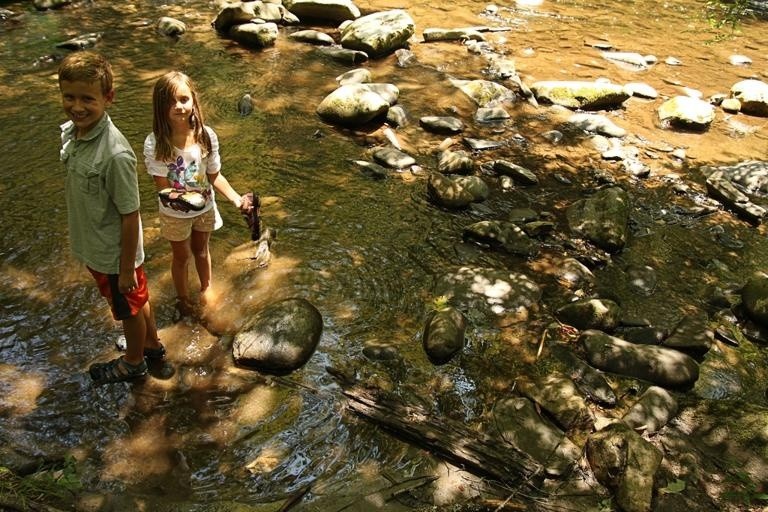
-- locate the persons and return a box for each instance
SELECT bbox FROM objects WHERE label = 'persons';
[58,50,165,380]
[143,71,262,298]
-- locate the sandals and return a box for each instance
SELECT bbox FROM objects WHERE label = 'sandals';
[157,187,205,212]
[240,191,262,241]
[89,334,165,384]
